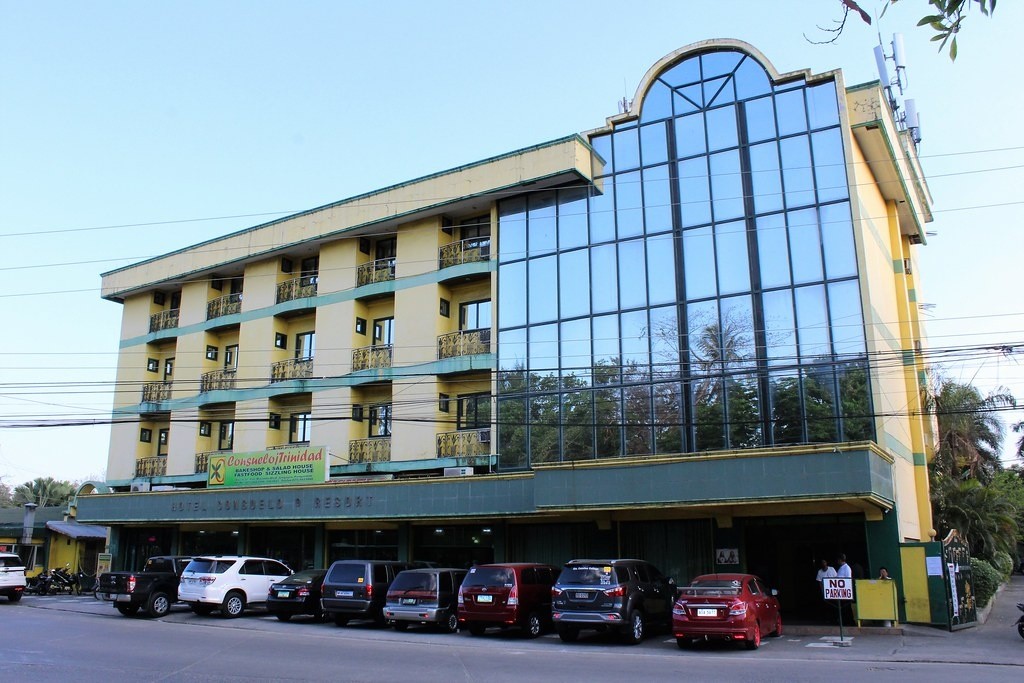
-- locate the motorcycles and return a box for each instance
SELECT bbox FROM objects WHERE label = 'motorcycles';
[24,563,100,601]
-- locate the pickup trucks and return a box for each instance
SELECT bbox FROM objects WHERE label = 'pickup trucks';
[99,555,196,617]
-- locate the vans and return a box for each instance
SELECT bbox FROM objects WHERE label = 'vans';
[318,560,418,628]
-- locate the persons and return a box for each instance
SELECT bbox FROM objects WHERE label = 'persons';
[877,567,892,581]
[718,551,726,563]
[815,558,839,622]
[835,553,856,627]
[727,551,737,563]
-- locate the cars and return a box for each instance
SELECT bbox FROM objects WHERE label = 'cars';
[266,568,331,625]
[672,573,782,650]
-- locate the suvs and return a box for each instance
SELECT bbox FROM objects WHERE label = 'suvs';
[177,555,297,619]
[383,568,473,636]
[459,563,563,638]
[0,552,27,603]
[550,558,678,645]
